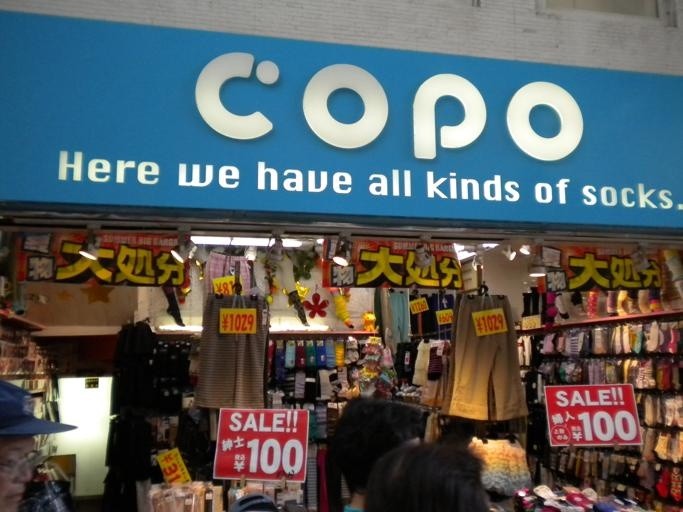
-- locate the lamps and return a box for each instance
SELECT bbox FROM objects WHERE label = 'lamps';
[414,234,433,270]
[267,229,289,262]
[169,226,198,263]
[501,236,549,279]
[78,229,102,260]
[331,231,354,269]
[628,245,651,273]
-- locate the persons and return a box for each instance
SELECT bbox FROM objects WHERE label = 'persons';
[0,380,78,512]
[356,436,497,512]
[329,392,430,510]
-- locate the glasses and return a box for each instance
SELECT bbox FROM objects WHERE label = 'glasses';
[0,450,45,477]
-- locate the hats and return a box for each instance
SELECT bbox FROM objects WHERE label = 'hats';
[0,378,78,441]
